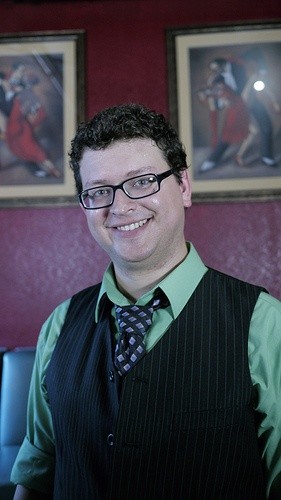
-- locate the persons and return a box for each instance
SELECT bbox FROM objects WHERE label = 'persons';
[197,57,276,172]
[10,104,281,500]
[0,63,64,180]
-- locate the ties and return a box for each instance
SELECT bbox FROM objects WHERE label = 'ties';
[113,293,170,377]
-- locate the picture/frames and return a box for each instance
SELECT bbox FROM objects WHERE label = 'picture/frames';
[163,21,281,203]
[0,30,86,208]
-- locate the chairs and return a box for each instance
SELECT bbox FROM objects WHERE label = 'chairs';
[0,352,35,486]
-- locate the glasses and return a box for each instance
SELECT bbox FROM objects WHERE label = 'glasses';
[75,168,177,209]
